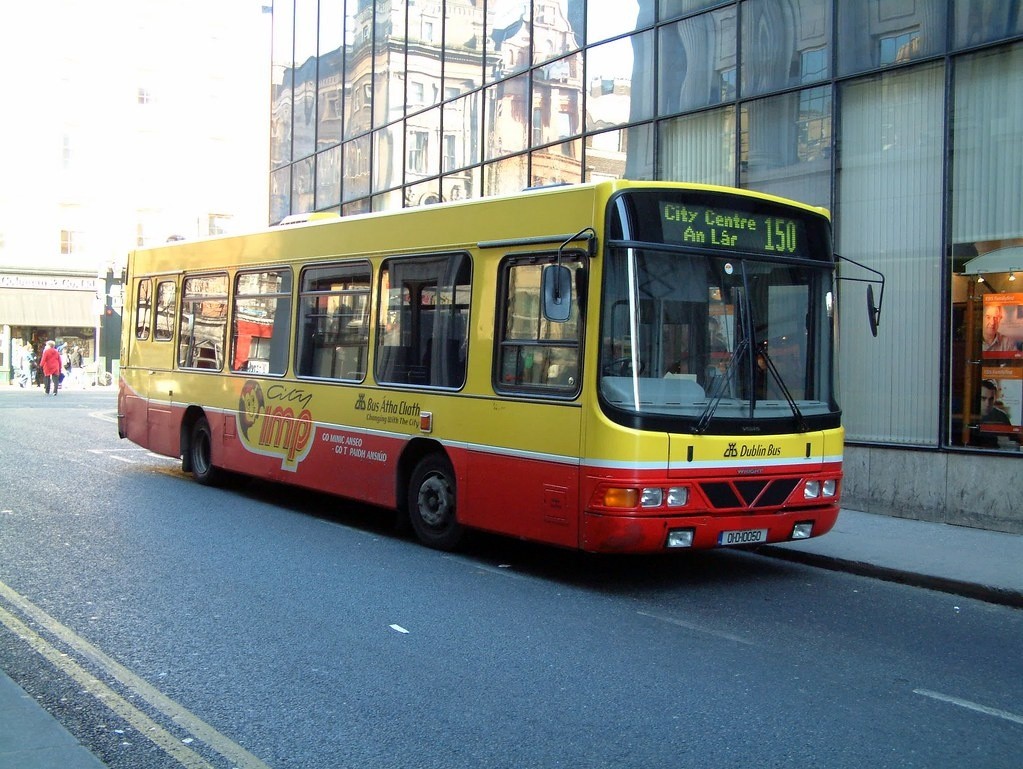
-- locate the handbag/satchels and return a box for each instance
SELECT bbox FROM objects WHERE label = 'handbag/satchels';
[59,373,65,383]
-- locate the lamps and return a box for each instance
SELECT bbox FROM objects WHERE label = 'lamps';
[977,269,989,282]
[1008,267,1020,282]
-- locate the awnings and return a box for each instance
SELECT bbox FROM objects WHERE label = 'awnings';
[0,289,98,328]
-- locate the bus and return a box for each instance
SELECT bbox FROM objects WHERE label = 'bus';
[116,179,886,557]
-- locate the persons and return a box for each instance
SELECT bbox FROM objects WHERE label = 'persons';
[708,314,732,352]
[18,340,86,396]
[982,305,1017,350]
[981,378,1011,425]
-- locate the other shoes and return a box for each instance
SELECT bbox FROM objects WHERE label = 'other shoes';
[44,390,49,394]
[53,393,57,396]
